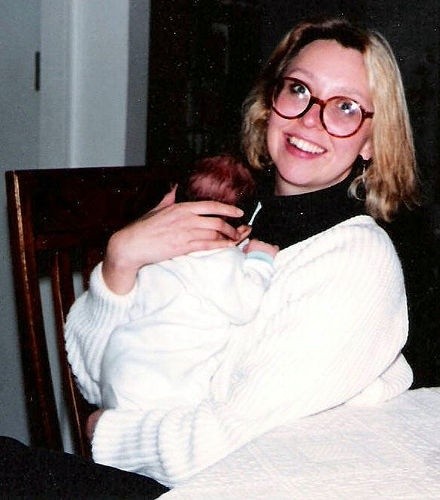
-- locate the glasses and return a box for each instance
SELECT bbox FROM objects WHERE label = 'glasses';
[269,76,375,139]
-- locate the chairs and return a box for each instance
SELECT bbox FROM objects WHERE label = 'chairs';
[5,166,177,457]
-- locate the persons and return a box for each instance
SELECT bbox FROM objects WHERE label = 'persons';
[100,158,278,418]
[1,18,419,499]
